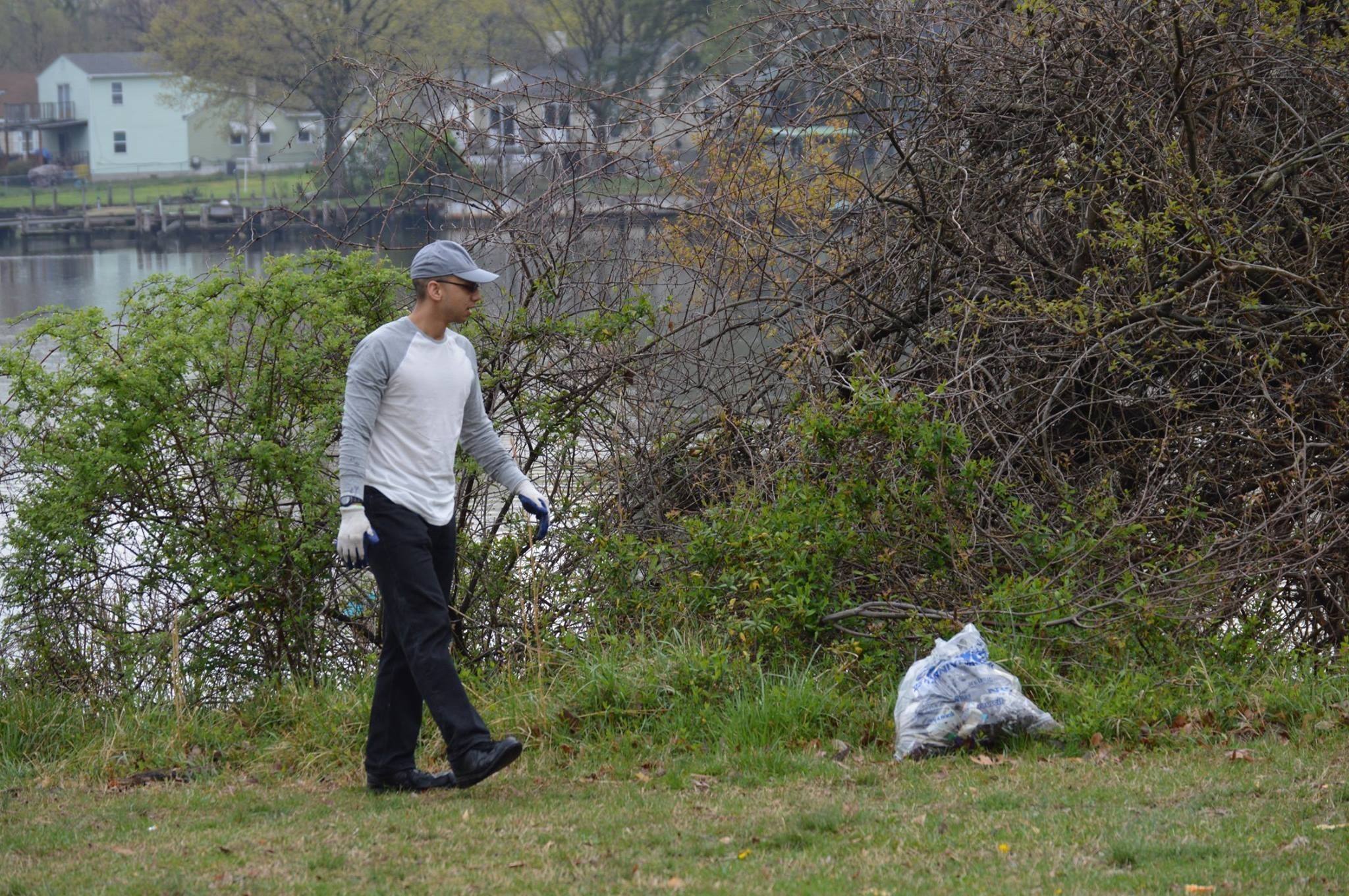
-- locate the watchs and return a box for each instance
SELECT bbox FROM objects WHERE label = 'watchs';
[340,495,363,507]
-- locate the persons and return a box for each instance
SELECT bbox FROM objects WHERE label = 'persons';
[31,147,52,164]
[335,239,549,790]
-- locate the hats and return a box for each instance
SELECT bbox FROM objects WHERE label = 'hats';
[409,240,500,283]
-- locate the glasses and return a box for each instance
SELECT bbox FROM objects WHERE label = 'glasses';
[436,279,477,293]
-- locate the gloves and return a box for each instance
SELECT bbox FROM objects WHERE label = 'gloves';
[515,481,551,540]
[335,504,379,570]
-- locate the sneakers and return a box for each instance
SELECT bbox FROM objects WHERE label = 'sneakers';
[451,737,523,788]
[366,767,455,792]
